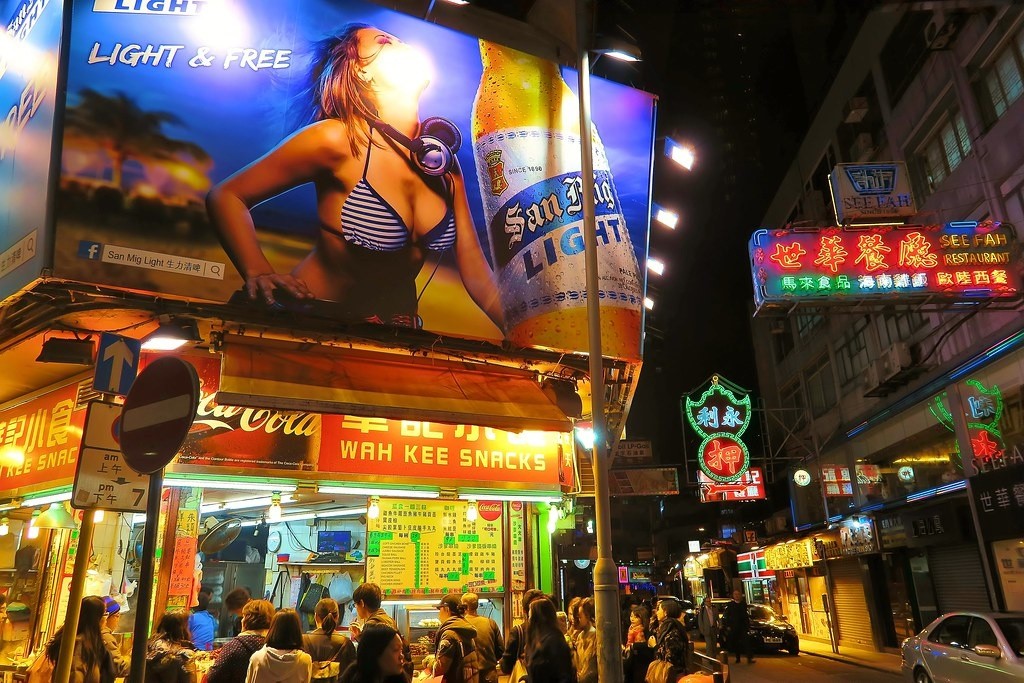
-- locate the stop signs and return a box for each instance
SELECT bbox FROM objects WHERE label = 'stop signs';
[117,356,197,471]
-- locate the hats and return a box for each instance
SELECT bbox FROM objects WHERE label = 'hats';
[432,594,461,607]
[101,595,120,617]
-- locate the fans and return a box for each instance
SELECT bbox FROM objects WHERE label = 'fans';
[199,515,243,555]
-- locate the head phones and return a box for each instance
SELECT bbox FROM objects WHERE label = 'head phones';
[366,108,462,176]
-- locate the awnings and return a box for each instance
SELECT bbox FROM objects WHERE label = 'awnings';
[215,341,574,434]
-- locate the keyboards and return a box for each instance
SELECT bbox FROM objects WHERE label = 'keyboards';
[315,557,345,563]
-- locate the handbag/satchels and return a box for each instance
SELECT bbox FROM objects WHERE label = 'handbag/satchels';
[269,570,353,632]
[645,652,673,683]
[475,645,497,672]
[507,624,529,683]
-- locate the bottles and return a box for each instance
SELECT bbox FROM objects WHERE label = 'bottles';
[470,39,641,360]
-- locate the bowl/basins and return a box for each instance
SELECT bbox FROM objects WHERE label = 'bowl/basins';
[277,554,289,561]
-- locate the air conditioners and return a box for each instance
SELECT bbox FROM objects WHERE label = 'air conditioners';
[924,9,956,48]
[856,341,911,397]
[848,135,874,161]
[802,190,825,223]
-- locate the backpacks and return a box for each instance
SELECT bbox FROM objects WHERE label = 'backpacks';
[396,629,414,681]
[305,635,349,683]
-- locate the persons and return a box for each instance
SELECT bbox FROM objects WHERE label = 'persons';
[145,610,197,683]
[621,596,690,683]
[202,23,505,335]
[207,583,414,683]
[26,596,130,683]
[0,594,7,623]
[422,589,598,683]
[188,586,217,650]
[698,590,753,663]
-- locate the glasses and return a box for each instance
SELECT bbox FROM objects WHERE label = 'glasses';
[100,612,109,618]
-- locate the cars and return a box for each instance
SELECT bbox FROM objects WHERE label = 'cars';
[656,595,799,655]
[900,611,1024,683]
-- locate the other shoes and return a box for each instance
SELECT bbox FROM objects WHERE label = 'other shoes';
[735,658,740,664]
[747,659,756,663]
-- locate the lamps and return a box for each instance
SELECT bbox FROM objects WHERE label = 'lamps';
[591,35,645,62]
[32,502,77,528]
[653,128,695,171]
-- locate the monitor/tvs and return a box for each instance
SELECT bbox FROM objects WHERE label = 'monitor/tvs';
[317,531,352,559]
